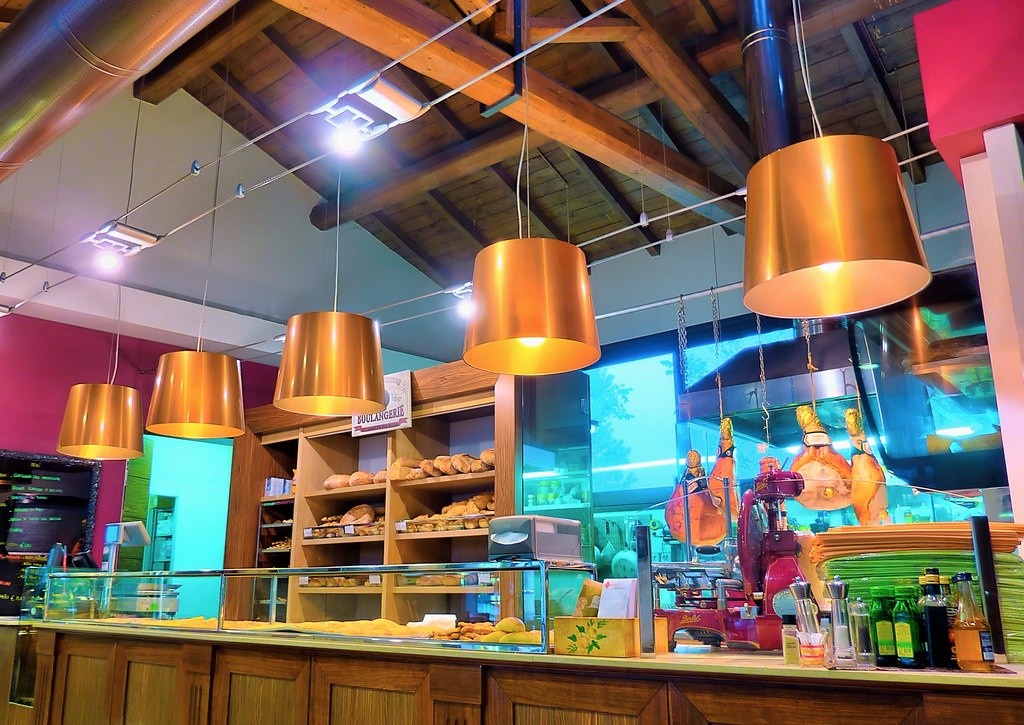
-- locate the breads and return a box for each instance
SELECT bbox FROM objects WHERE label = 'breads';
[312,448,496,538]
[415,575,554,645]
[303,577,383,587]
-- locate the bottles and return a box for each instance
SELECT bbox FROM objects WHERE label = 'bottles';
[778,567,996,672]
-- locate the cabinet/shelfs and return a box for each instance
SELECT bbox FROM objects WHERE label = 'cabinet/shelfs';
[219,359,595,623]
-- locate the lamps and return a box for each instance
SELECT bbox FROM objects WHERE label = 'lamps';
[272,150,386,417]
[55,99,144,460]
[743,0,932,318]
[463,0,601,375]
[145,32,247,439]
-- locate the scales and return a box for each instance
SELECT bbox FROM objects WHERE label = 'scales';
[98,521,182,618]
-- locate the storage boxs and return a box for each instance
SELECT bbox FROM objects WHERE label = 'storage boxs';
[553,616,668,659]
